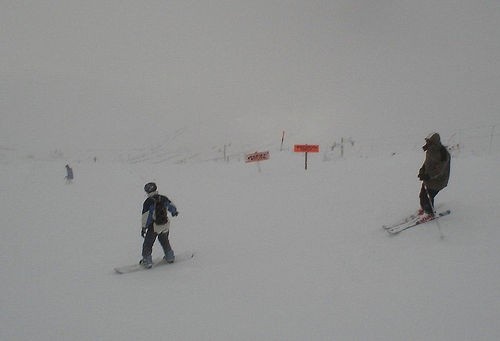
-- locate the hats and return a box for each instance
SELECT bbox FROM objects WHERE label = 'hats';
[144,182,157,192]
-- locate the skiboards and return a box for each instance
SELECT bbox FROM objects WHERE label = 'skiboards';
[383,207,450,235]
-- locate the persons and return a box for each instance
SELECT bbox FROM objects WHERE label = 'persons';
[65,164,73,184]
[417,132,451,223]
[139,183,178,269]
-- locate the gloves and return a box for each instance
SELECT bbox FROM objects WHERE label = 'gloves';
[172,211,178,216]
[141,227,147,237]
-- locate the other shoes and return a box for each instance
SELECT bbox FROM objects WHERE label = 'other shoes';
[140,259,152,268]
[163,255,174,263]
[422,212,433,221]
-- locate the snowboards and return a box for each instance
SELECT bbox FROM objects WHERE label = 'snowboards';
[115,251,195,276]
